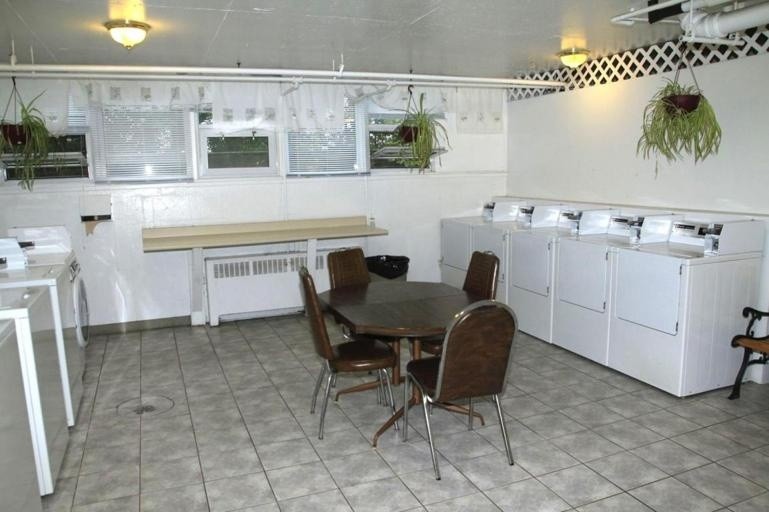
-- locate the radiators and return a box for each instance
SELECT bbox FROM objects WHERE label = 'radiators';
[206,250,337,327]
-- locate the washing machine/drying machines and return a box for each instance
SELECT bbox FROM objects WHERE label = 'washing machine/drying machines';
[1,225,90,512]
[441,196,767,399]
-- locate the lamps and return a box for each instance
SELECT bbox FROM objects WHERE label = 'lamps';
[104,20,152,49]
[556,48,591,69]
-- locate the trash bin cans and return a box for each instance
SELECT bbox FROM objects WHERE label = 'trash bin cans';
[365,255,409,283]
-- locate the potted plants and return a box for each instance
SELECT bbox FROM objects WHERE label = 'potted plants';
[0,89,64,192]
[635,76,722,179]
[387,92,452,175]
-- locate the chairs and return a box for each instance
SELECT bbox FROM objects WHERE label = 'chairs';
[298,265,399,441]
[400,297,517,480]
[327,246,372,341]
[409,248,503,361]
[728,307,769,400]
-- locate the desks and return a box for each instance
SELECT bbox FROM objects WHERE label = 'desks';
[313,280,487,448]
